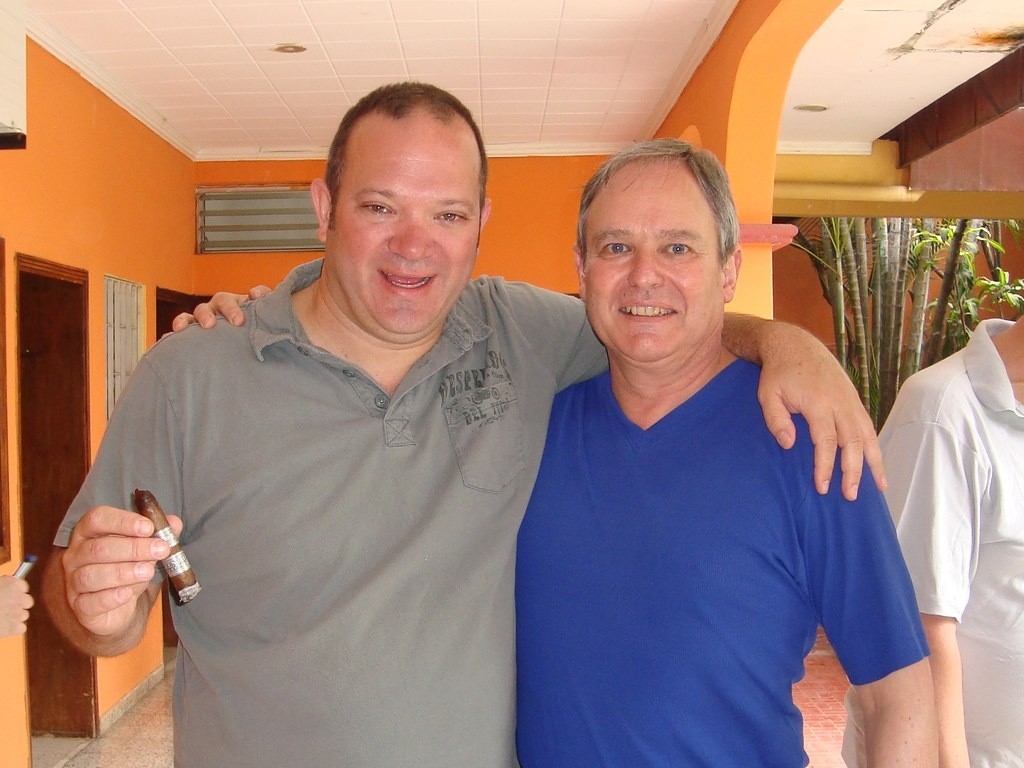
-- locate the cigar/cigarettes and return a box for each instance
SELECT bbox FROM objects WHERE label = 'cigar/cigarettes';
[135,486,201,603]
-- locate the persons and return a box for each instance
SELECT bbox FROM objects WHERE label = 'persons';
[839,313,1024,768]
[39,81,890,768]
[156,139,941,767]
